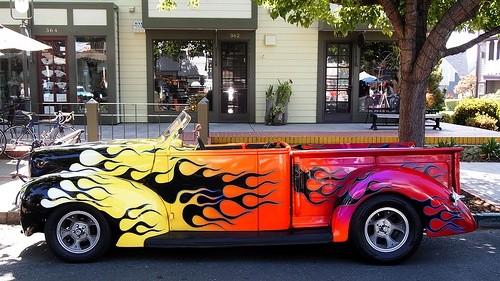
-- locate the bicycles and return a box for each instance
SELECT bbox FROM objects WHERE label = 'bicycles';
[1,110,82,161]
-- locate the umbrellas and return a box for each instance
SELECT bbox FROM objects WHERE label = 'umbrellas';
[42,44,107,65]
[0,24,53,80]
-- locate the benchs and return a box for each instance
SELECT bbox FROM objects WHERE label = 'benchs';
[369,107,442,132]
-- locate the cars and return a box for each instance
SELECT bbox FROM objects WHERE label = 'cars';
[19,111,477,263]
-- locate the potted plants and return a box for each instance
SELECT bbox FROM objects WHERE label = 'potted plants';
[265,79,293,125]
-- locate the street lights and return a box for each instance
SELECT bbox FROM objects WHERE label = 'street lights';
[10,0,33,101]
